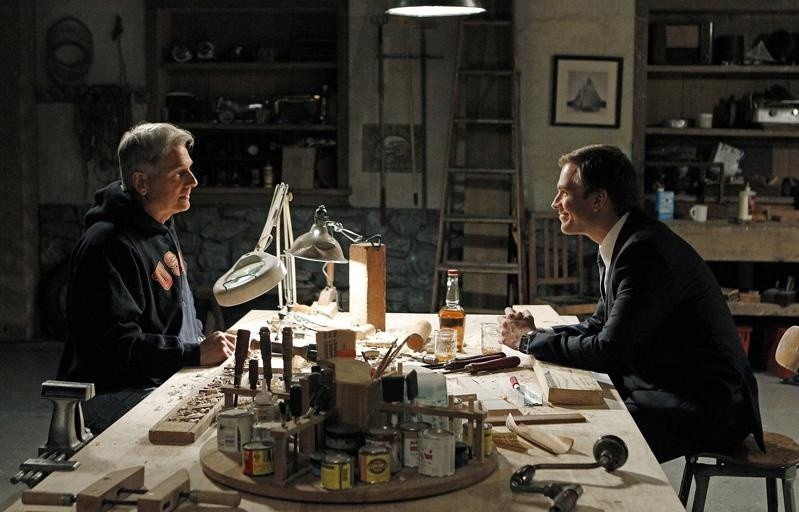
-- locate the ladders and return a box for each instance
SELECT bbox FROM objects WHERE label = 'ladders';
[428,0,529,313]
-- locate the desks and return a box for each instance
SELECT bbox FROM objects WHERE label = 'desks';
[1,315,687,512]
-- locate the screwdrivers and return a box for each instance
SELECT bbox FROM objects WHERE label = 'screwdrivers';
[258,326,275,393]
[232,326,252,408]
[21,488,139,508]
[119,486,243,507]
[248,358,258,403]
[421,351,521,376]
[280,325,293,410]
[510,377,524,389]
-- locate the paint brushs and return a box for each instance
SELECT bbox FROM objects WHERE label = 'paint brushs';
[372,335,411,379]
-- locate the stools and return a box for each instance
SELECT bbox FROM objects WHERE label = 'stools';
[680,430,799,511]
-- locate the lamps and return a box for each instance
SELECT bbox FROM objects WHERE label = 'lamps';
[385,1,486,21]
[208,183,297,308]
[282,204,389,331]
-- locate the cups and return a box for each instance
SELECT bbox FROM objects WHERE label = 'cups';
[688,204,708,222]
[434,327,458,363]
[479,320,503,355]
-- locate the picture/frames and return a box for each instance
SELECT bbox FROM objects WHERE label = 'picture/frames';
[549,54,624,129]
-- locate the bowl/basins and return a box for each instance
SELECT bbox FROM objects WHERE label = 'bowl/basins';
[664,117,695,128]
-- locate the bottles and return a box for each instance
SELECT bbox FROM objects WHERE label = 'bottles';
[738,181,757,221]
[439,267,466,355]
[694,112,712,129]
[250,378,273,421]
[264,166,273,189]
[250,169,260,185]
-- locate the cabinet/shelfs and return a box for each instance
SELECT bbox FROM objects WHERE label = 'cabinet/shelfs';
[641,0,798,262]
[152,0,348,193]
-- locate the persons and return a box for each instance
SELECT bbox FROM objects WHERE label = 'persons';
[497,143,765,466]
[57,121,237,436]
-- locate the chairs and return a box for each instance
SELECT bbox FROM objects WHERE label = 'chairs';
[527,213,601,316]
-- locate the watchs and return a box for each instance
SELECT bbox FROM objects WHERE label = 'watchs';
[518,330,538,354]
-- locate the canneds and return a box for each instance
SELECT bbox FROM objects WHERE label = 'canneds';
[240,440,275,477]
[461,421,494,459]
[308,420,458,492]
